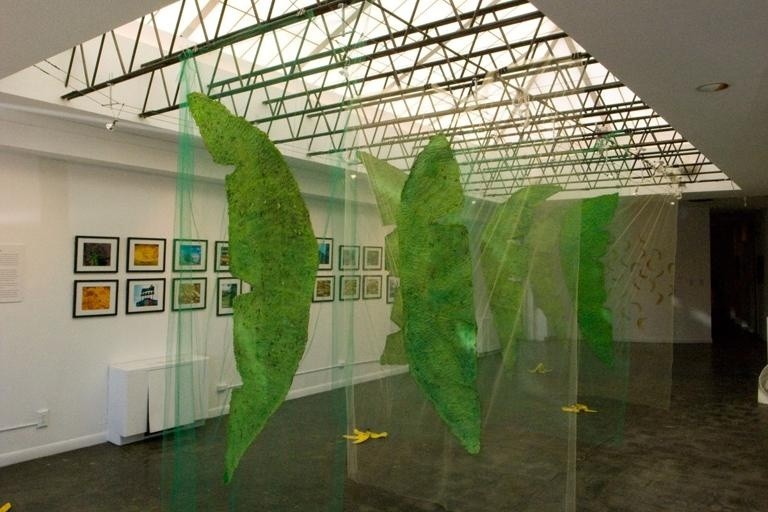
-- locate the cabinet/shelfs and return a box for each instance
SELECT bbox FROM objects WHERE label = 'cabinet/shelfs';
[105,353,209,446]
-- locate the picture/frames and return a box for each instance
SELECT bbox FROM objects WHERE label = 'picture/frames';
[125,277,167,314]
[71,233,121,275]
[171,237,209,274]
[214,239,230,273]
[71,278,121,318]
[215,276,243,317]
[171,276,209,312]
[311,234,401,305]
[125,236,167,274]
